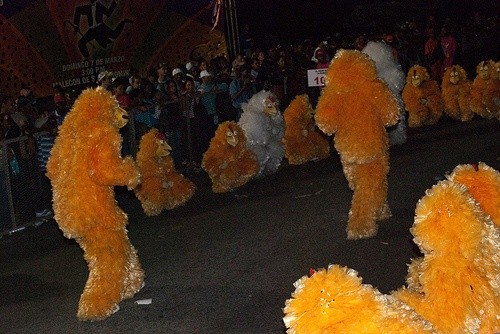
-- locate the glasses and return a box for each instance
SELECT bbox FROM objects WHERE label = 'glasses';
[134,81,142,84]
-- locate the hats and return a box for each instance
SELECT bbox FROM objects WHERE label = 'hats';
[158,63,167,69]
[199,70,211,78]
[20,89,32,96]
[98,71,112,81]
[186,62,194,71]
[172,68,182,76]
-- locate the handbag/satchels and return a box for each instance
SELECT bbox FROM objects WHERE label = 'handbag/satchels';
[9,147,19,176]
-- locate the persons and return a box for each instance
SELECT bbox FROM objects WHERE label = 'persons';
[0,11,500,230]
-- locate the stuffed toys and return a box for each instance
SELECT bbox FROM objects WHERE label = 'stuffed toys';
[283,94,330,165]
[282,264,437,334]
[361,40,407,146]
[46,85,146,322]
[444,161,500,229]
[237,89,286,178]
[467,60,500,120]
[134,128,197,216]
[391,182,500,334]
[441,64,475,122]
[314,49,401,240]
[402,65,443,127]
[200,121,259,193]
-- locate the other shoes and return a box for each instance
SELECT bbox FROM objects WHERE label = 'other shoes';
[192,167,203,172]
[135,280,146,294]
[180,158,194,167]
[114,304,120,314]
[36,209,52,218]
